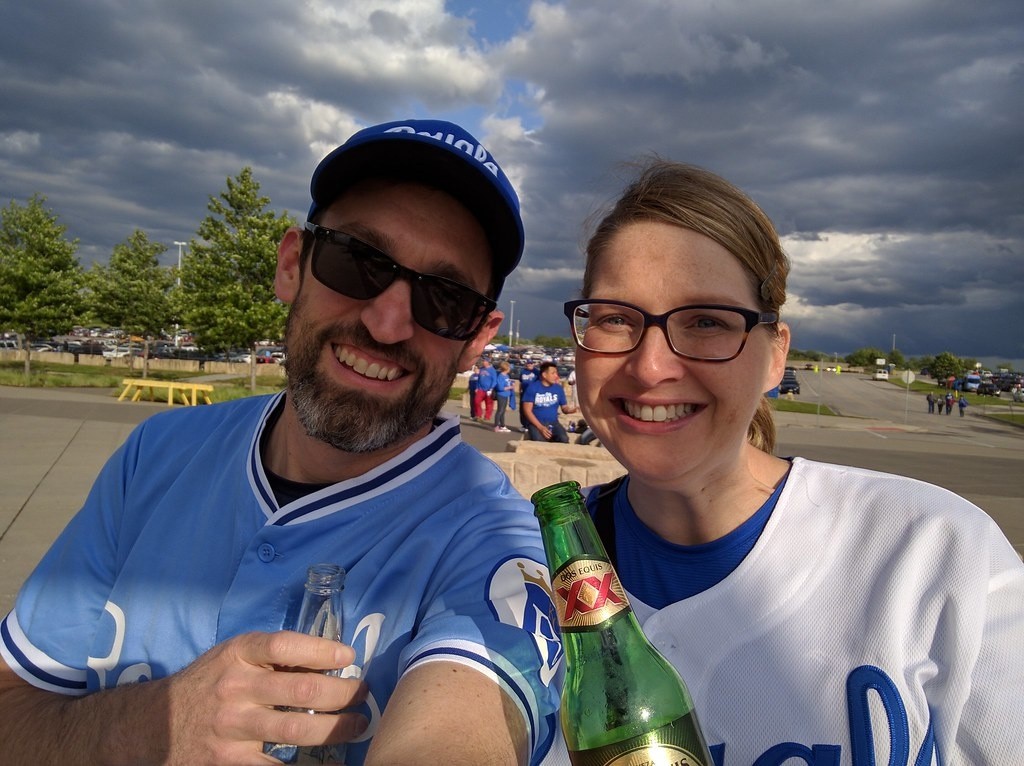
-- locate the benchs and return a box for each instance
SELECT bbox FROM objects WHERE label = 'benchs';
[117,379,214,407]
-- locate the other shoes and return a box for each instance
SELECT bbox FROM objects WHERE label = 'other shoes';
[519,425,528,433]
[495,426,511,433]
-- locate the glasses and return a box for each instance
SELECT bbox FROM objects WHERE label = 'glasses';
[305,221,496,341]
[564,298,777,362]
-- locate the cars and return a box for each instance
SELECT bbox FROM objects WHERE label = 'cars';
[920,368,1024,398]
[0,325,288,366]
[780,366,800,394]
[872,368,889,381]
[805,364,814,370]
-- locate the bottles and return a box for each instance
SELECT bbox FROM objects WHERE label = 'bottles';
[263,564,345,766]
[531,481,713,766]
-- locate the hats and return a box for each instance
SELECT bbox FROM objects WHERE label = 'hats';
[305,119,524,301]
[526,359,533,363]
[482,357,491,363]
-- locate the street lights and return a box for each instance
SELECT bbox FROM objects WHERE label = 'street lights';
[172,240,187,349]
[508,300,516,347]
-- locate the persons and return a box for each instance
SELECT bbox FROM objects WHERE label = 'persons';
[531,158,1024,766]
[1,120,567,766]
[938,399,944,415]
[945,392,954,415]
[521,362,581,443]
[959,395,969,417]
[568,371,579,406]
[519,359,538,432]
[927,392,936,414]
[467,357,516,432]
[580,428,604,447]
